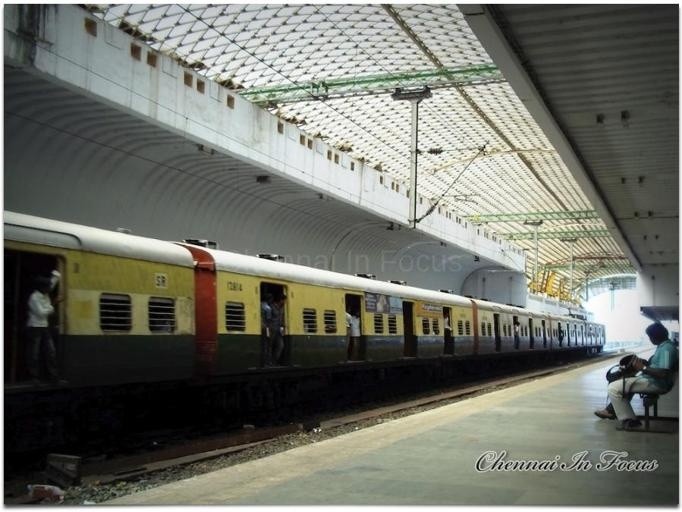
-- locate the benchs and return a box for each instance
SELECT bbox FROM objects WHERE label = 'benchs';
[623,392,679,435]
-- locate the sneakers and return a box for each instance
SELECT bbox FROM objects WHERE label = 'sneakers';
[614,419,643,430]
[594,409,616,420]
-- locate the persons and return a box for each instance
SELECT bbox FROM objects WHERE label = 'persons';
[344,303,353,361]
[269,292,288,366]
[45,287,63,371]
[593,321,679,431]
[21,271,68,386]
[443,310,454,354]
[260,291,274,367]
[349,309,363,361]
[558,325,564,346]
[514,321,521,349]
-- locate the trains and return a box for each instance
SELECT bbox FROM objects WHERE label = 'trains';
[4,210,605,453]
